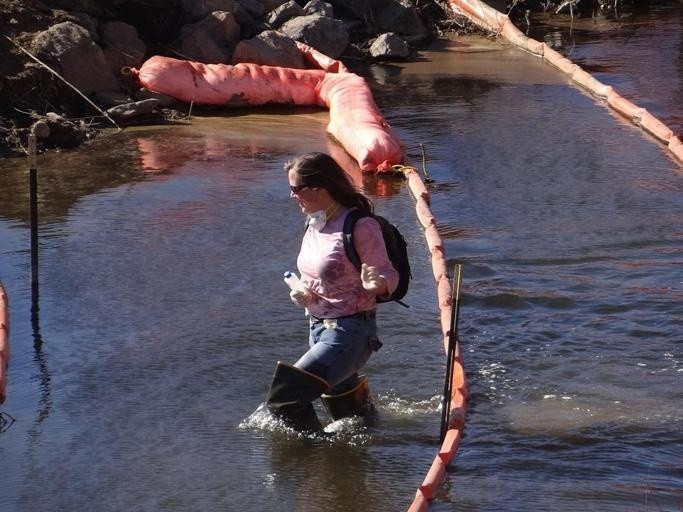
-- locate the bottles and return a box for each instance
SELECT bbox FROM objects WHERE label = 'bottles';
[283,271,313,304]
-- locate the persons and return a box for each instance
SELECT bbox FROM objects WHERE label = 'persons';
[266,151,400,435]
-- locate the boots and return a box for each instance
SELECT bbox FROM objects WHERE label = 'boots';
[320,374,378,422]
[265,360,333,435]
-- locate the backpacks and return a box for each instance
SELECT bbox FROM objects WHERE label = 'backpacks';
[304,210,410,303]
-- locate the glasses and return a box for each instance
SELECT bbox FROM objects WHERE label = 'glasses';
[288,183,311,195]
